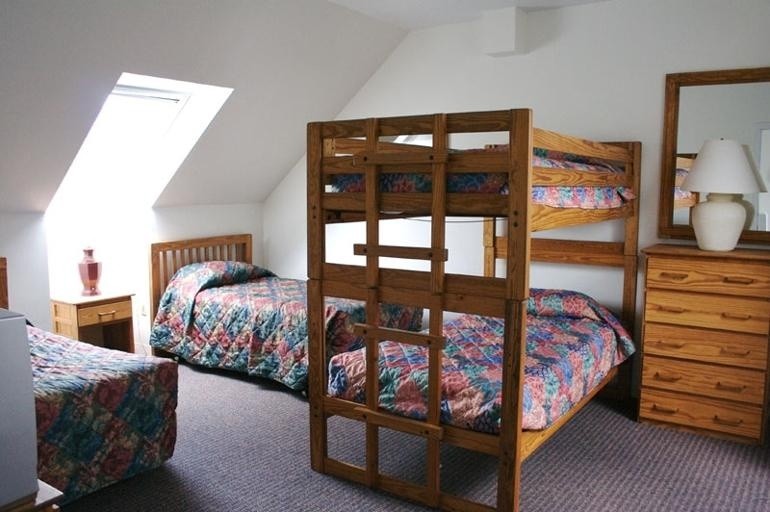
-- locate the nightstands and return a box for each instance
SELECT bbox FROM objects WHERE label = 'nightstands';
[638,245,769,445]
[49,292,135,355]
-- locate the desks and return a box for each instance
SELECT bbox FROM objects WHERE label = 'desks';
[1,479,66,511]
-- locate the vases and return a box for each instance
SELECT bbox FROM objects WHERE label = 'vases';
[78,250,101,295]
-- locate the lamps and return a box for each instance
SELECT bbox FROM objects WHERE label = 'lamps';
[681,138,761,252]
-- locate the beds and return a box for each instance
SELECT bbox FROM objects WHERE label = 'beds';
[154,233,424,391]
[0,256,179,507]
[306,108,641,512]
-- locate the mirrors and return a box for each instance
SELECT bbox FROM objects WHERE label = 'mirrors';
[658,67,770,246]
